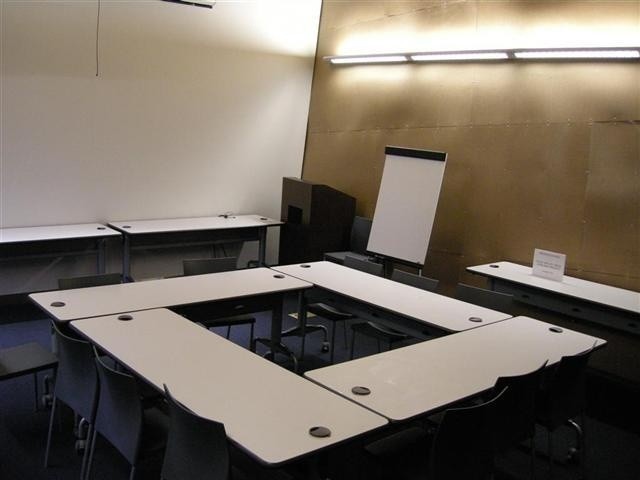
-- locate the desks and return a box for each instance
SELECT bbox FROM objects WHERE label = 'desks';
[303,316,604,465]
[272,261,511,333]
[0,214,284,283]
[464,261,639,334]
[26,266,315,407]
[68,308,389,467]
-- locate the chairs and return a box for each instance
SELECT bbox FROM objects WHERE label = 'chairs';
[455,283,515,315]
[86,350,167,476]
[59,272,126,289]
[437,383,518,470]
[363,423,432,474]
[300,258,383,365]
[37,321,101,477]
[160,384,228,476]
[351,269,441,364]
[541,338,600,468]
[183,259,257,351]
[491,357,552,467]
